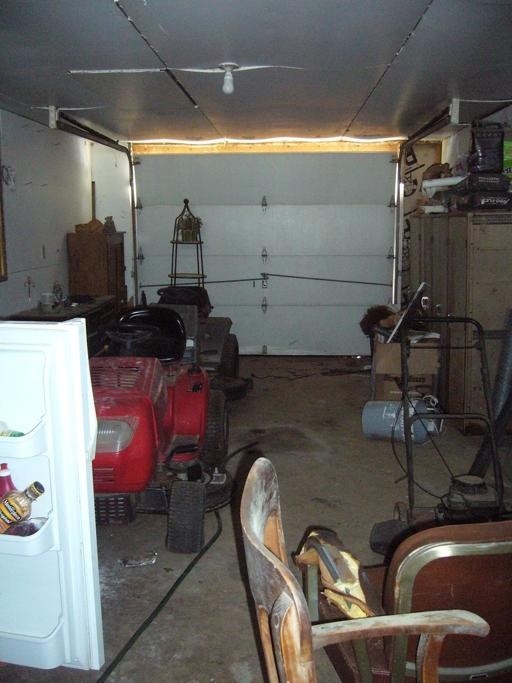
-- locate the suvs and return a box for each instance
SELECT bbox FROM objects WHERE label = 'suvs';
[87,305,230,554]
[145,286,238,385]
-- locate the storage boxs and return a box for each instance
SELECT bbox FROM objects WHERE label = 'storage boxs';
[372,341,441,402]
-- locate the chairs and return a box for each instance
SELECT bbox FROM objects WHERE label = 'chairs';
[303,507,512,680]
[238,455,490,681]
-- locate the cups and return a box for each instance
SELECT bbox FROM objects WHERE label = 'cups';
[39,291,57,314]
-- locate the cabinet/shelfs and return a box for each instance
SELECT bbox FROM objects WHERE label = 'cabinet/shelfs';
[430,210,511,435]
[5,291,120,358]
[163,194,209,286]
[64,229,128,299]
[407,214,430,334]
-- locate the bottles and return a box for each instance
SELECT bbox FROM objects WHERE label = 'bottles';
[1,480,45,534]
[0,461,18,535]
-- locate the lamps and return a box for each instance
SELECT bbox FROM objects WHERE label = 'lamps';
[218,60,241,93]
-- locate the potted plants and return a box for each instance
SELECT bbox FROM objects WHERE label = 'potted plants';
[175,215,203,240]
